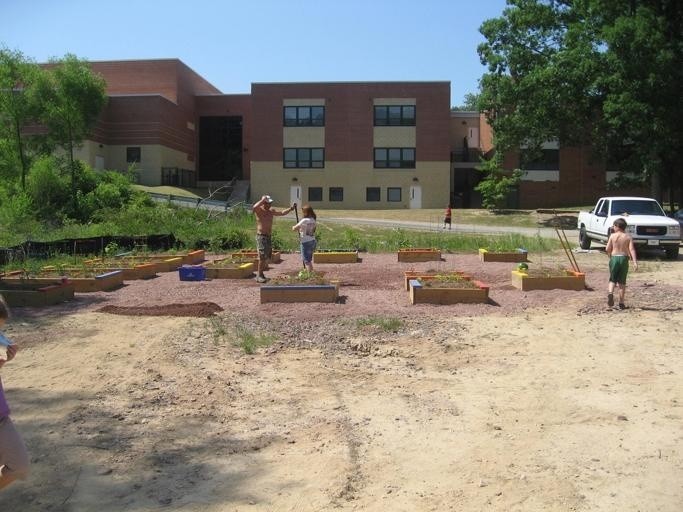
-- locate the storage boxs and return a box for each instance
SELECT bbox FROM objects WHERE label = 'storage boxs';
[177,264,207,281]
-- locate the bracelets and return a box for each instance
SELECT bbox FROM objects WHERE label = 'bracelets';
[290,205,294,211]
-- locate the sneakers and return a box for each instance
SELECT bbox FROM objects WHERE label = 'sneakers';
[607,292,614,306]
[617,302,625,311]
[255,274,270,282]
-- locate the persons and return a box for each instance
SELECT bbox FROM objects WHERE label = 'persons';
[0,295,30,492]
[604,219,637,309]
[251,194,296,283]
[292,204,316,273]
[442,203,451,230]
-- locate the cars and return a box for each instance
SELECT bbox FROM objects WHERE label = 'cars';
[667,208,682,241]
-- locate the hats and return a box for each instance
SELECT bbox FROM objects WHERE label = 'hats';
[261,194,273,204]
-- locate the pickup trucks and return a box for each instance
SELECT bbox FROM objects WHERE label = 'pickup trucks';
[577,196,681,259]
